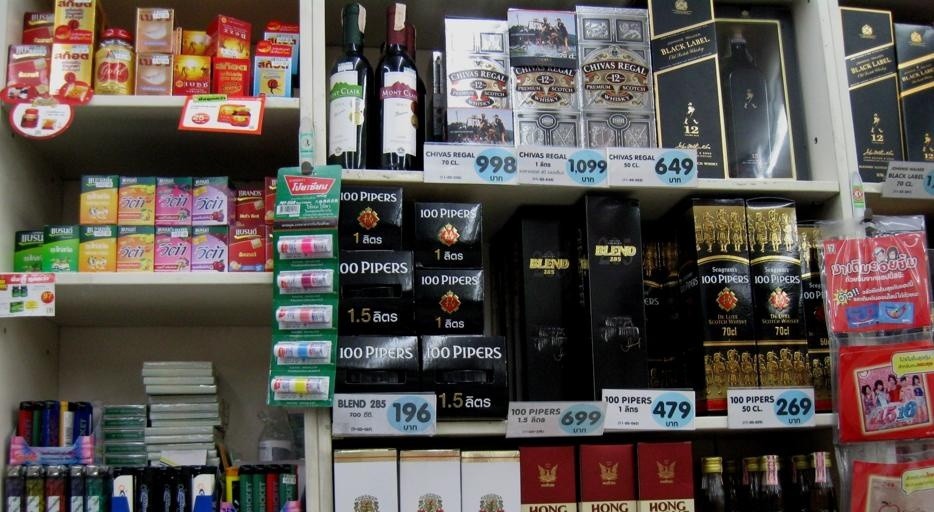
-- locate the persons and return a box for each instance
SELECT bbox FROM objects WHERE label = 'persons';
[457,113,507,144]
[862,373,929,432]
[521,15,572,52]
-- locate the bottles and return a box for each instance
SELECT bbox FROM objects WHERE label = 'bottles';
[328,2,425,170]
[257,409,294,463]
[698,450,839,510]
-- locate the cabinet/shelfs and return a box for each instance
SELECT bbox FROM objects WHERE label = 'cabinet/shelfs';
[0,0,934,512]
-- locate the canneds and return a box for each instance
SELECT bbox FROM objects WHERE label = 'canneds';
[93,28,135,95]
